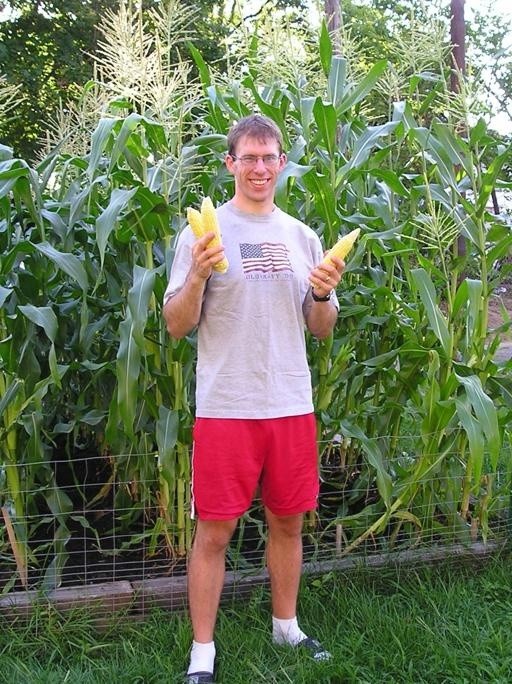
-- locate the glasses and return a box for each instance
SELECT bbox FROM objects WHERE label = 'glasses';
[229,153,283,165]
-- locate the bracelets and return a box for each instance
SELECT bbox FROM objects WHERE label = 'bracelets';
[309,285,335,304]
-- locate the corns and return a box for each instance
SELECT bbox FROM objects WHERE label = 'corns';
[309,228,361,289]
[187,196,230,274]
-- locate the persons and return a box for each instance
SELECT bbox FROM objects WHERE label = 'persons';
[159,112,352,683]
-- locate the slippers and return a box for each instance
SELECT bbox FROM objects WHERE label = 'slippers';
[185,646,217,683]
[276,637,335,662]
